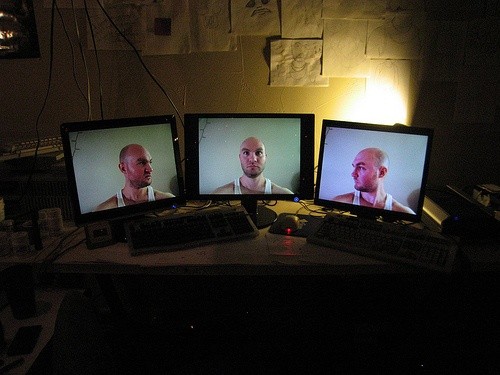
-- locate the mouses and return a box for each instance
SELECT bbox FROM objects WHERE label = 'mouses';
[280,215,299,234]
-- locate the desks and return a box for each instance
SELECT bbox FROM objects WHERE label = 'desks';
[0,198,500,308]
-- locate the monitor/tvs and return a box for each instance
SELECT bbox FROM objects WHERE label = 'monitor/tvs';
[60,115,186,244]
[184,112,314,229]
[314,119,434,223]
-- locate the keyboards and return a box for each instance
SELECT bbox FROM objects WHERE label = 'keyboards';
[125,205,259,255]
[306,212,460,274]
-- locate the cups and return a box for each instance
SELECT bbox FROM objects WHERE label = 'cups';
[38,208,64,247]
[0,220,31,257]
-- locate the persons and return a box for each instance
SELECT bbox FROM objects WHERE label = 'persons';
[94,144,175,212]
[332,148,416,215]
[211,137,294,194]
